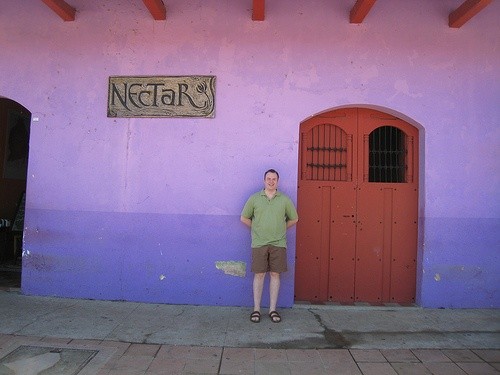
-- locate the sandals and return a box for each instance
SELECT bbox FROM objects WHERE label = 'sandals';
[251,311,260,323]
[269,311,280,323]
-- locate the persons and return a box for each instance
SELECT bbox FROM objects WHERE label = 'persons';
[239,168,300,324]
